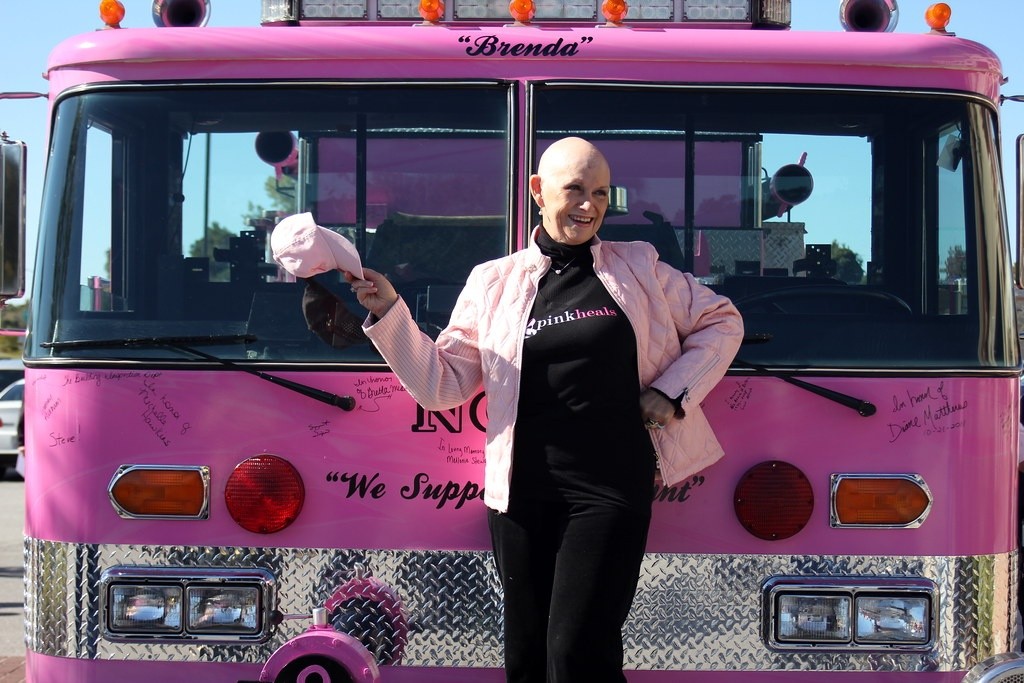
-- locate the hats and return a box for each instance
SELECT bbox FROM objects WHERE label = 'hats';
[271,211,366,281]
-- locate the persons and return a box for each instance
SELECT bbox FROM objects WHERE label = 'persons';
[344,135,745,683]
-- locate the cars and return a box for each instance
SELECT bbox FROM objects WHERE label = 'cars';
[0,363,24,482]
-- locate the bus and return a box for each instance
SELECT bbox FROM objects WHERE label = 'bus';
[0,0,1024,683]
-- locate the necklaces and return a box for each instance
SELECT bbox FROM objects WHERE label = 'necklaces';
[550,253,580,274]
